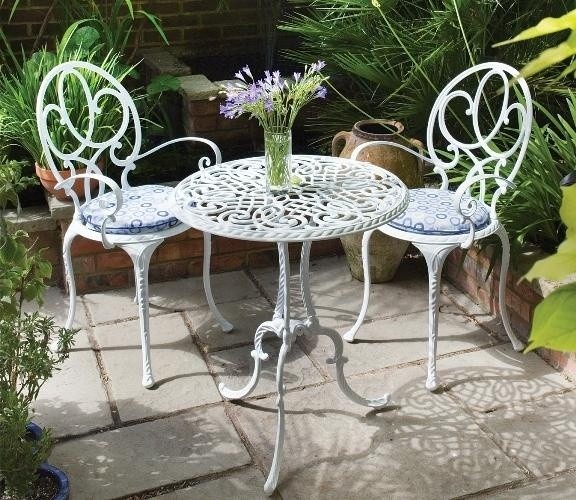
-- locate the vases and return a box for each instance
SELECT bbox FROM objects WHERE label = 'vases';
[331,117,424,285]
[263,126,293,193]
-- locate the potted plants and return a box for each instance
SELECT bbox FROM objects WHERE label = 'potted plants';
[0,38,165,200]
[0,315,82,500]
[1,214,53,461]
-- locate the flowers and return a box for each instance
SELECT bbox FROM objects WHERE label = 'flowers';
[219,58,330,185]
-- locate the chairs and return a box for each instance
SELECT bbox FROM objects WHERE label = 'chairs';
[342,62,526,391]
[36,61,234,387]
